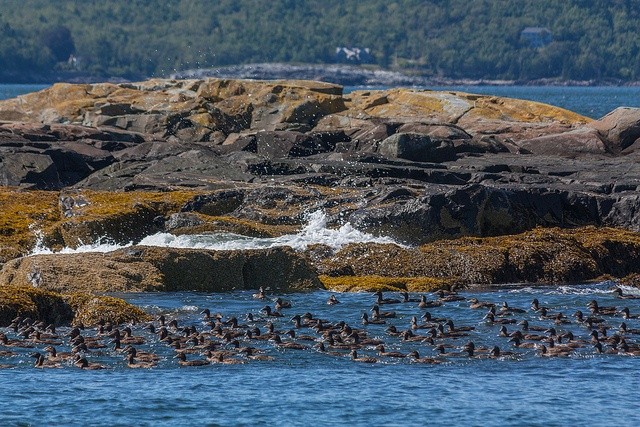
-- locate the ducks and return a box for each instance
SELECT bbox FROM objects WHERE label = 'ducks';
[0,288,639,373]
